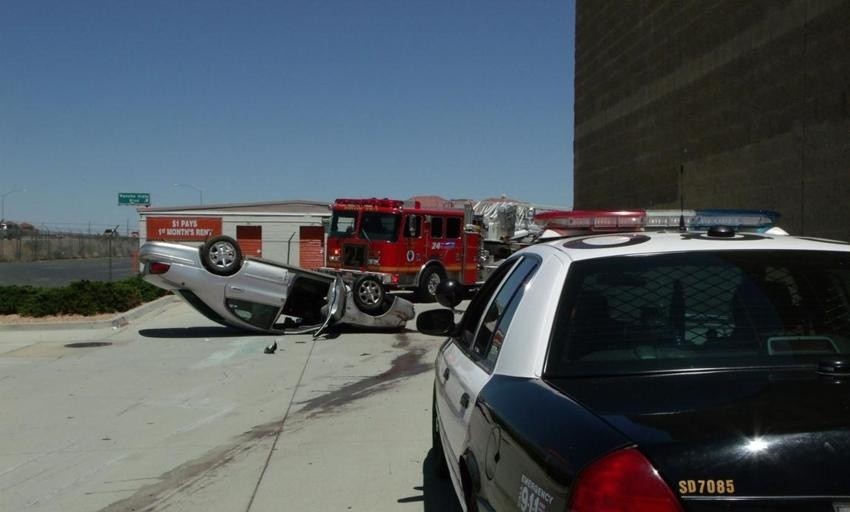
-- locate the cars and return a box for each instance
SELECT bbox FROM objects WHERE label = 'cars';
[104,229,118,235]
[138,235,416,338]
[416,207,850,512]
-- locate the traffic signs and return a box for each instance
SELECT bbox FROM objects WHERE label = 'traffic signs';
[118,192,149,206]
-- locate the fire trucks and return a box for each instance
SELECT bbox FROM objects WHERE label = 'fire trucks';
[321,197,490,301]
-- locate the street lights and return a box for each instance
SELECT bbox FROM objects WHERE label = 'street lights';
[1,186,28,225]
[173,183,205,207]
